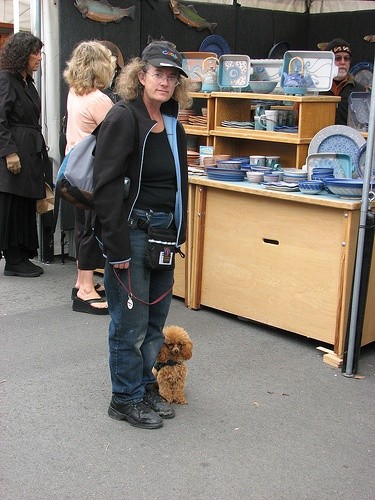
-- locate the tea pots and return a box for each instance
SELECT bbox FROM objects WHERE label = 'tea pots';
[194,57,219,93]
[282,56,308,96]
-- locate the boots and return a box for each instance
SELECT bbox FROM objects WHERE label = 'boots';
[3,246,40,277]
[19,249,44,274]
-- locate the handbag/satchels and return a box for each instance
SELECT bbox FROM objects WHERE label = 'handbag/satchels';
[48,121,131,235]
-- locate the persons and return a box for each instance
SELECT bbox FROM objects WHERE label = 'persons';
[319,39,366,126]
[64,42,115,313]
[0,31,53,277]
[93,42,188,429]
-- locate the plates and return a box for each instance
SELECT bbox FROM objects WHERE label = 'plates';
[259,182,298,191]
[221,120,254,129]
[177,109,207,127]
[349,61,374,91]
[179,35,338,92]
[187,150,200,167]
[307,125,375,180]
[206,166,248,181]
[280,168,308,183]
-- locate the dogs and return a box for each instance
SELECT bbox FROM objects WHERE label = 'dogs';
[151,325,193,405]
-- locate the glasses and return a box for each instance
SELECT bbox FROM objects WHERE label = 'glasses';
[335,55,353,61]
[144,70,178,81]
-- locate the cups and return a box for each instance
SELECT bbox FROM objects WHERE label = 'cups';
[259,110,278,131]
[255,105,296,126]
[188,82,202,92]
[272,172,283,180]
[249,155,280,168]
[250,164,280,173]
[201,108,207,116]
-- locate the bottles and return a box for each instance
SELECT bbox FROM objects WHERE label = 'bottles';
[311,167,334,195]
[254,115,264,130]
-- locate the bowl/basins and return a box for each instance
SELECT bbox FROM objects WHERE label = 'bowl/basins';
[249,80,278,93]
[215,161,242,170]
[323,178,375,200]
[298,180,324,194]
[246,172,279,183]
[214,155,249,168]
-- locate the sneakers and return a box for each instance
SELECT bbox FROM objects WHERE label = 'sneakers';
[107,394,164,430]
[144,384,176,419]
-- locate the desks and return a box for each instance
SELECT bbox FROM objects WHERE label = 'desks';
[171,172,375,359]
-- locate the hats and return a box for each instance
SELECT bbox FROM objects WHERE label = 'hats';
[323,38,353,57]
[141,41,189,79]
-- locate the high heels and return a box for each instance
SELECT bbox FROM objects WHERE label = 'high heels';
[71,295,110,316]
[71,282,106,300]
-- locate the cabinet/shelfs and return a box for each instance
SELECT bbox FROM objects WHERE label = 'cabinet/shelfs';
[211,90,343,169]
[177,92,216,148]
